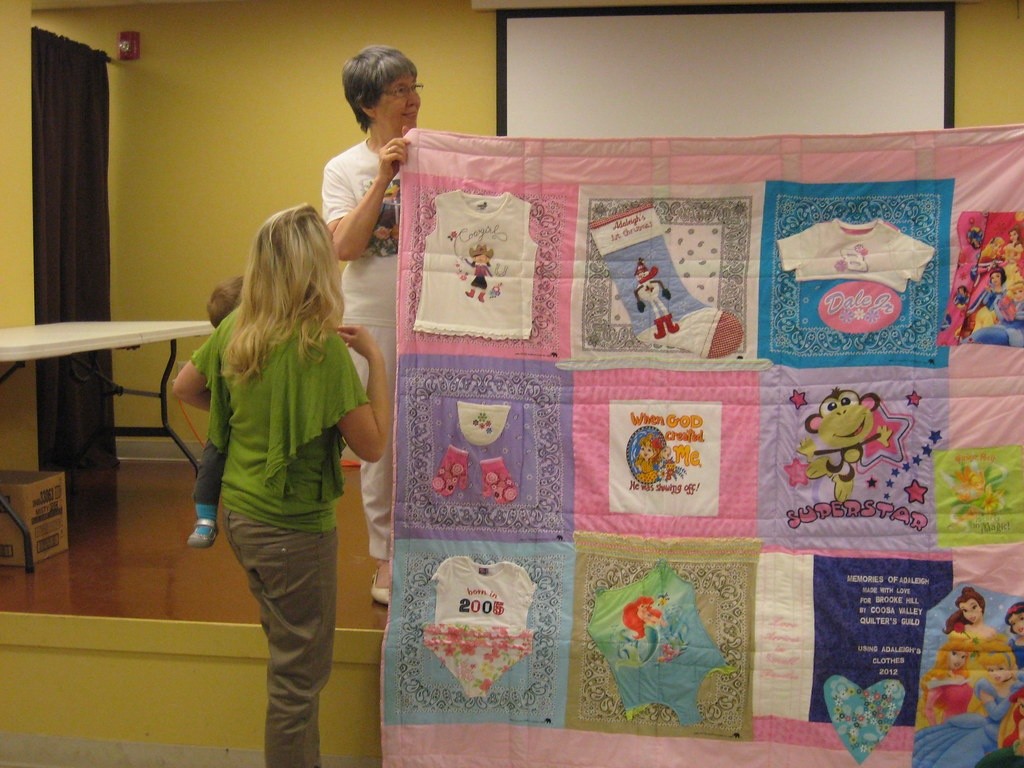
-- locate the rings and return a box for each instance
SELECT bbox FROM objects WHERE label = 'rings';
[387,147,391,153]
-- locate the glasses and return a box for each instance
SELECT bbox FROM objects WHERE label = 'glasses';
[380,84,424,98]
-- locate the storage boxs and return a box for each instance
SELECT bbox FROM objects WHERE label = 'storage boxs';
[0,469,69,567]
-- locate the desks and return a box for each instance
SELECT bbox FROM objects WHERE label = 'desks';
[0,320,216,572]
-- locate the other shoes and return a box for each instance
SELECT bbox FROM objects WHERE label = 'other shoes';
[187,518,217,547]
[371,570,390,606]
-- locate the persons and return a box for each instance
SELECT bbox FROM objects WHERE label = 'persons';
[320,42,421,606]
[171,204,389,768]
